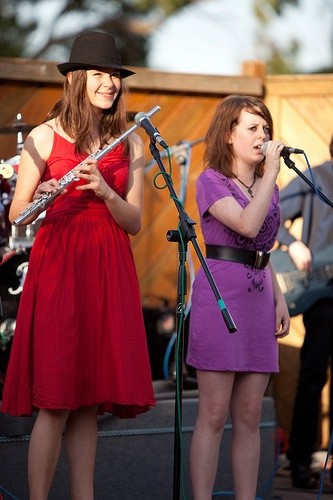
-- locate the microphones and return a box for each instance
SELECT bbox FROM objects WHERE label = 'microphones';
[134,112,168,149]
[261,142,304,155]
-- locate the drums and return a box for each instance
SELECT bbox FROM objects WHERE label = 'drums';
[0,237,30,318]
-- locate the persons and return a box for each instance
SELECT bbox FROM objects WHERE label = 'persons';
[0,31,154,500]
[276,134,333,487]
[185,95,290,500]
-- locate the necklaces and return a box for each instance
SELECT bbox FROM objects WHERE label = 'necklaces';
[231,174,256,198]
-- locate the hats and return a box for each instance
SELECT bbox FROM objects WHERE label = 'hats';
[56,31,136,79]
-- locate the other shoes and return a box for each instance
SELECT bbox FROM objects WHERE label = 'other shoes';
[291,467,320,490]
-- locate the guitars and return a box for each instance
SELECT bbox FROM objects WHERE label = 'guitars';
[270,242,333,320]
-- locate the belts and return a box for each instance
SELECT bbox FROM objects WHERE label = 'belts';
[205,244,271,270]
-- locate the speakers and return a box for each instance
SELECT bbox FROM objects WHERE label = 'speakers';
[141,306,190,381]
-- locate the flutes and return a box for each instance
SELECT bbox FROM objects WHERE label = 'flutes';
[12,106,162,227]
